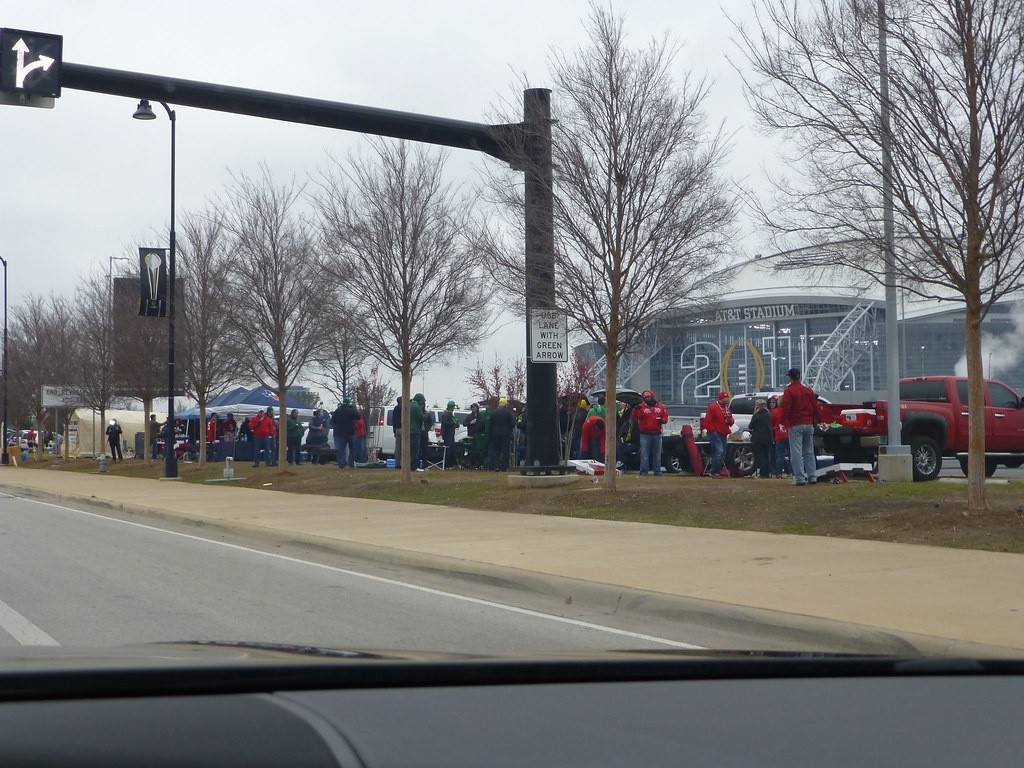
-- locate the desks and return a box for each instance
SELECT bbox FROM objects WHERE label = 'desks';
[427,444,459,470]
[695,441,775,479]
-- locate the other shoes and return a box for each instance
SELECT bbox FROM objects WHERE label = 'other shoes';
[287,461,303,466]
[336,464,347,469]
[759,476,770,479]
[253,462,259,468]
[310,459,324,465]
[109,458,124,464]
[708,470,722,479]
[265,459,278,466]
[775,474,785,479]
[485,466,508,472]
[639,470,649,478]
[415,468,431,472]
[795,480,817,486]
[654,470,664,476]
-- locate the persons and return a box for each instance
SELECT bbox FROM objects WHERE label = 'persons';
[27,427,62,454]
[779,369,820,485]
[105,418,124,461]
[704,390,735,479]
[748,394,821,479]
[392,390,669,477]
[149,397,368,469]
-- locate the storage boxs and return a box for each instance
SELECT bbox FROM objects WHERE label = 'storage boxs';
[840,409,877,430]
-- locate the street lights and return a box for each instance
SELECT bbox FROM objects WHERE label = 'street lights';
[131,94,181,480]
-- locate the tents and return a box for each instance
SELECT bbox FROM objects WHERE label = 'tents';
[63,408,169,458]
[172,385,314,463]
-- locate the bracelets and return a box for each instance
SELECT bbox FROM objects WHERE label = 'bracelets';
[779,425,784,427]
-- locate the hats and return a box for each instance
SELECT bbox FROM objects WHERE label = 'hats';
[498,397,507,405]
[643,390,653,398]
[413,393,424,401]
[267,407,273,412]
[718,392,729,400]
[578,400,586,407]
[447,401,455,408]
[343,397,351,404]
[471,403,478,407]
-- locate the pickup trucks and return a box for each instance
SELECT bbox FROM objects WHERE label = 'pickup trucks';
[817,374,1024,480]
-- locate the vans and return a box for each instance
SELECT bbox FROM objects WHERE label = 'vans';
[726,393,830,437]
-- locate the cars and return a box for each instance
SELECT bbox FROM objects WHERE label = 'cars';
[5,428,67,453]
[364,404,489,459]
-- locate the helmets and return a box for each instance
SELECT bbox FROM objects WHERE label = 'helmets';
[257,406,265,413]
